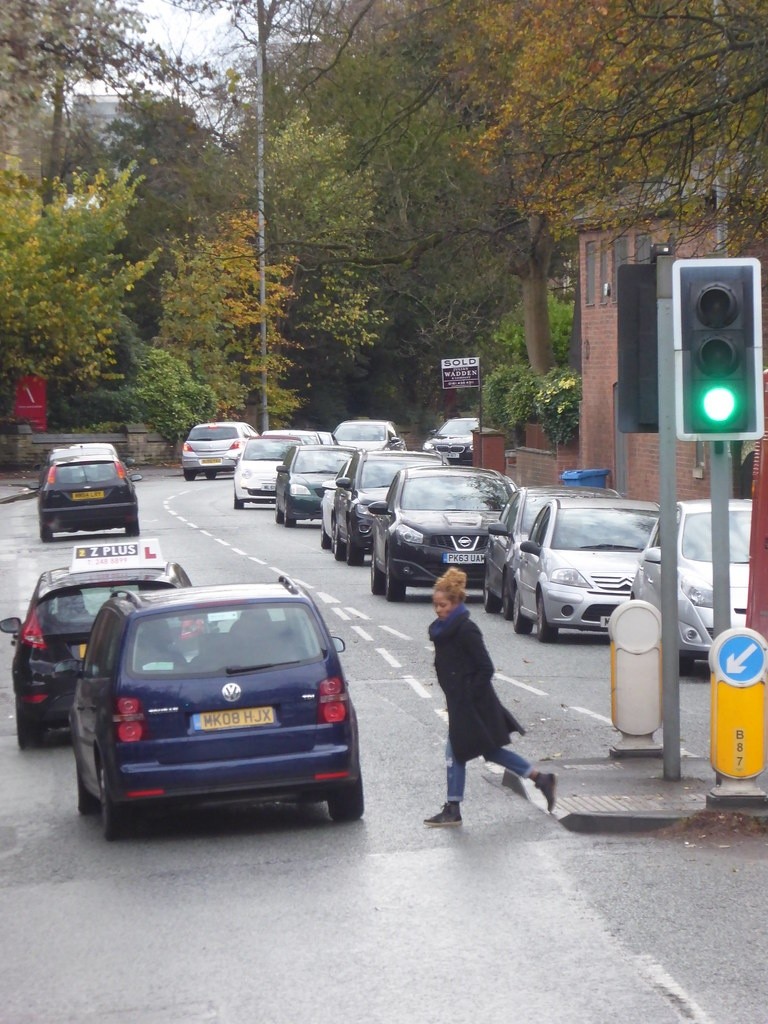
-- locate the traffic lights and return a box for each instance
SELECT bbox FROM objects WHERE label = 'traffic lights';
[671,257,765,441]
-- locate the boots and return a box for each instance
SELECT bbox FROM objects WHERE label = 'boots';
[535,772,555,812]
[424,802,464,826]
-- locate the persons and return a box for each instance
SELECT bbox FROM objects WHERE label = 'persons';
[424,566,557,825]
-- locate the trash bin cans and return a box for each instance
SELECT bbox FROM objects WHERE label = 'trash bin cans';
[560,468,611,489]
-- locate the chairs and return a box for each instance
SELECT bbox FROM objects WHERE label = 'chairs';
[135,631,185,671]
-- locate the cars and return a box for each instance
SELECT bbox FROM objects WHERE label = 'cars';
[262,428,340,446]
[182,421,260,481]
[483,484,626,621]
[334,419,408,452]
[29,441,143,542]
[274,445,365,528]
[366,465,518,601]
[513,498,660,644]
[226,436,309,508]
[423,416,482,466]
[320,458,355,549]
[0,537,219,751]
[332,451,448,565]
[628,499,756,676]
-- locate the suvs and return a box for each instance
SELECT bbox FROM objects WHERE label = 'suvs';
[51,574,364,841]
